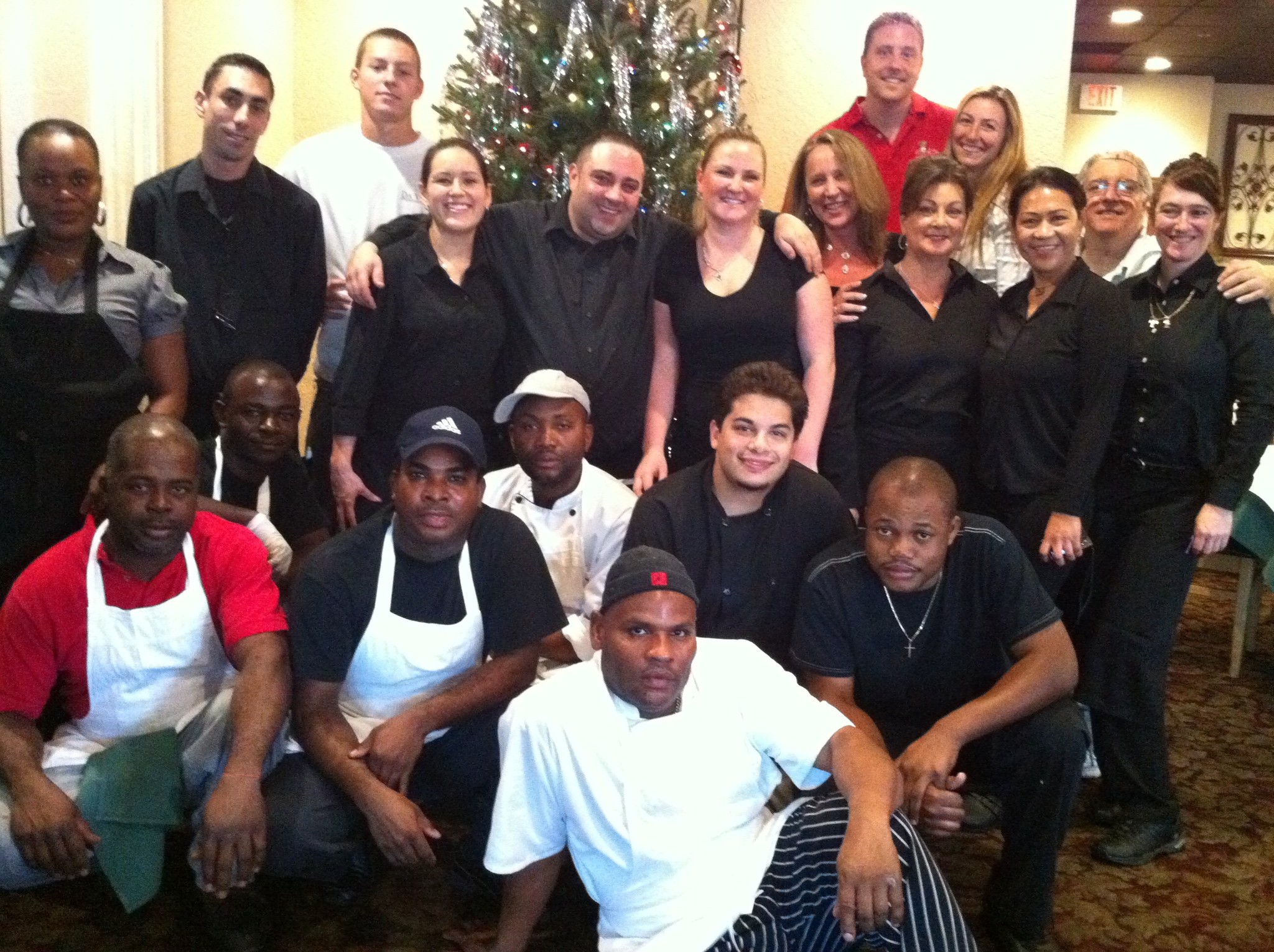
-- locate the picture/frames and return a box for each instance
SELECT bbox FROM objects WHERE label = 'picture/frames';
[1214,110,1274,258]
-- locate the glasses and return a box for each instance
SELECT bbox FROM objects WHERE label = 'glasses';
[1085,179,1149,197]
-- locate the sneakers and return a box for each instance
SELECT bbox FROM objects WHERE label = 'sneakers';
[958,791,1005,832]
[1081,799,1132,828]
[1091,817,1186,866]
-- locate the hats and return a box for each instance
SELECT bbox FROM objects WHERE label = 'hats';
[394,406,489,474]
[493,368,591,423]
[600,544,701,613]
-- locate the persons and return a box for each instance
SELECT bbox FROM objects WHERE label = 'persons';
[461,366,641,697]
[1062,147,1274,304]
[264,402,578,950]
[123,52,331,441]
[791,458,1080,951]
[272,29,441,464]
[773,129,914,329]
[1,415,314,951]
[939,80,1037,297]
[780,8,958,243]
[180,361,355,610]
[816,149,1007,837]
[990,163,1129,833]
[484,543,984,949]
[629,127,841,496]
[342,127,828,489]
[0,118,188,618]
[1078,153,1273,865]
[613,358,862,693]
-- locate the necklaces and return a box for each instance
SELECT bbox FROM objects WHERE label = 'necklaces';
[1033,278,1065,297]
[897,262,943,311]
[36,242,84,269]
[699,233,742,283]
[437,255,466,286]
[1147,289,1198,330]
[882,567,944,658]
[823,238,866,276]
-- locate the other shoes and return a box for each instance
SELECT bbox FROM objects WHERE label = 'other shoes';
[320,846,373,902]
